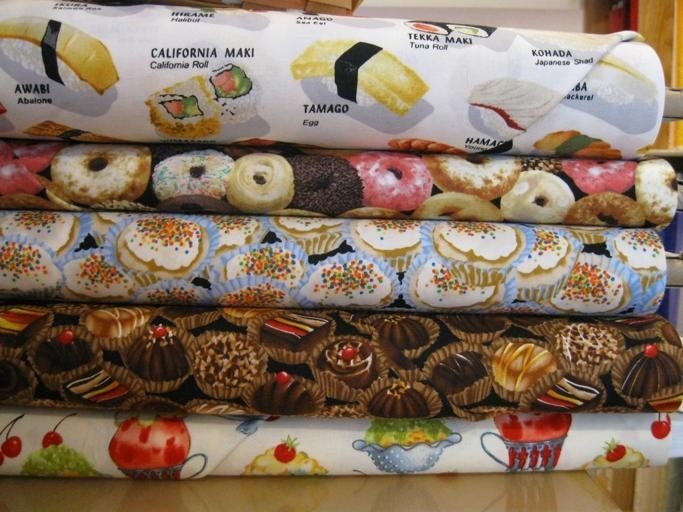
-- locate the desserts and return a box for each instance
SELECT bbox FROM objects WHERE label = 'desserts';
[0,213,682,414]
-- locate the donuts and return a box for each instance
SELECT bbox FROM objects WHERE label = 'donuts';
[0,139,678,227]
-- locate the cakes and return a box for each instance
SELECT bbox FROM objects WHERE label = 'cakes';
[109,412,190,470]
[580,438,647,469]
[19,445,94,478]
[353,417,463,447]
[493,412,571,441]
[239,434,329,477]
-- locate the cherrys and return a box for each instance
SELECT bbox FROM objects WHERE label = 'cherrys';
[1,413,25,467]
[650,413,670,439]
[42,414,77,447]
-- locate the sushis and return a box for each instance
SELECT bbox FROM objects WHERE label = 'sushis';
[291,39,429,116]
[468,77,565,138]
[0,15,120,95]
[534,131,622,160]
[205,60,262,126]
[24,120,126,142]
[144,76,220,142]
